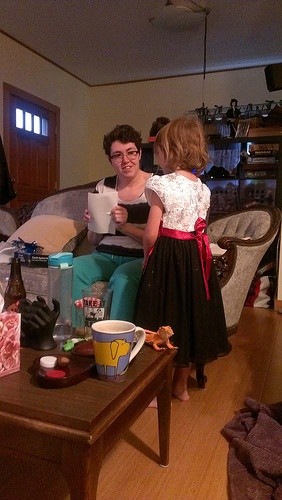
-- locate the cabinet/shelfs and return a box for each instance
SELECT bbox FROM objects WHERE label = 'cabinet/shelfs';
[140,137,282,217]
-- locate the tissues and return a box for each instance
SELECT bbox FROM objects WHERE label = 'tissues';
[0,292,22,378]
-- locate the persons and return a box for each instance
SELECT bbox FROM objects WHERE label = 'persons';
[134,116,232,408]
[20,296,60,350]
[226,99,242,137]
[61,125,154,327]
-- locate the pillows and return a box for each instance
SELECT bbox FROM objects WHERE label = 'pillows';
[8,215,87,263]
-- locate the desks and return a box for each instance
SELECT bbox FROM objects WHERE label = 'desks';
[0,322,176,500]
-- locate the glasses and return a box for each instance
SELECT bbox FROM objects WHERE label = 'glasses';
[111,147,141,160]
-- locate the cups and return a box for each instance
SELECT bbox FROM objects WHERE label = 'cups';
[81,290,114,341]
[91,320,147,382]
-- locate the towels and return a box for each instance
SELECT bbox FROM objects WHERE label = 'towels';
[218,396,282,500]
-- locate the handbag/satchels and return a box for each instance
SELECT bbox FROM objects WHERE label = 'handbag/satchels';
[244,261,276,309]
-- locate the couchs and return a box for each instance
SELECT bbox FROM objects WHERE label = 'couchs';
[0,180,280,336]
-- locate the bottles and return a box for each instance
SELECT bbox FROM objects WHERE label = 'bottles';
[47,252,74,342]
[4,257,26,347]
